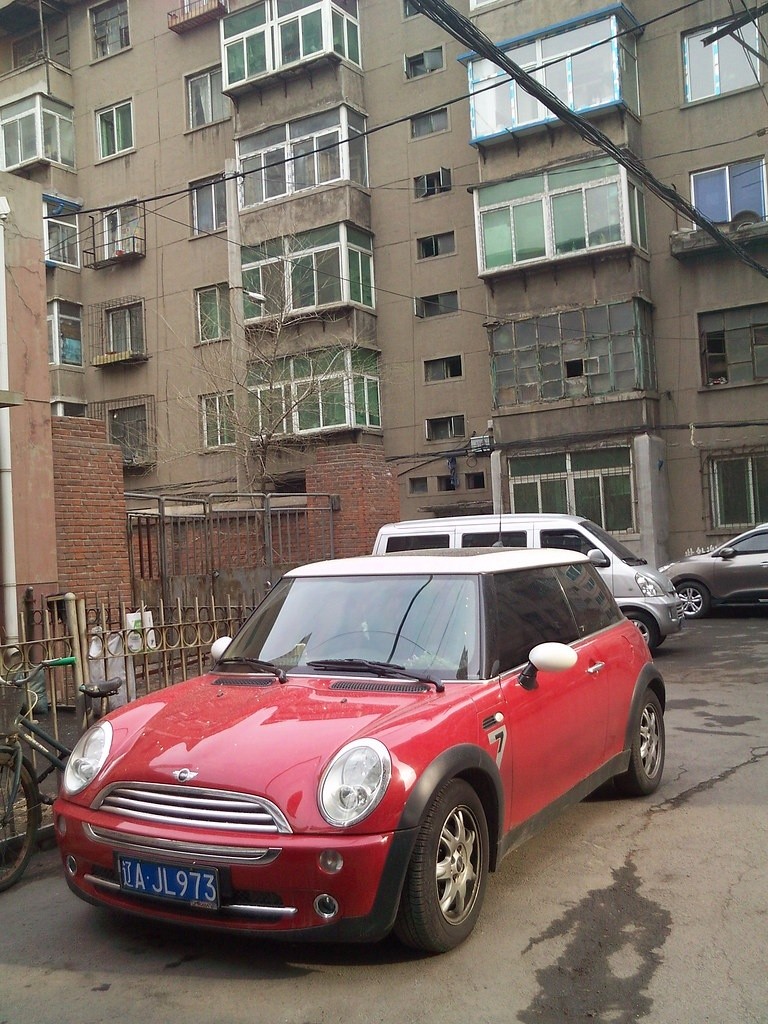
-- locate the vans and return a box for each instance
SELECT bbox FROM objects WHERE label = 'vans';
[365,512,686,657]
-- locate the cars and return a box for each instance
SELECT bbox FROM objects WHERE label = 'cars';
[51,544,669,958]
[659,521,768,617]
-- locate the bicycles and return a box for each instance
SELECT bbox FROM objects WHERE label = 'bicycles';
[0,655,120,895]
